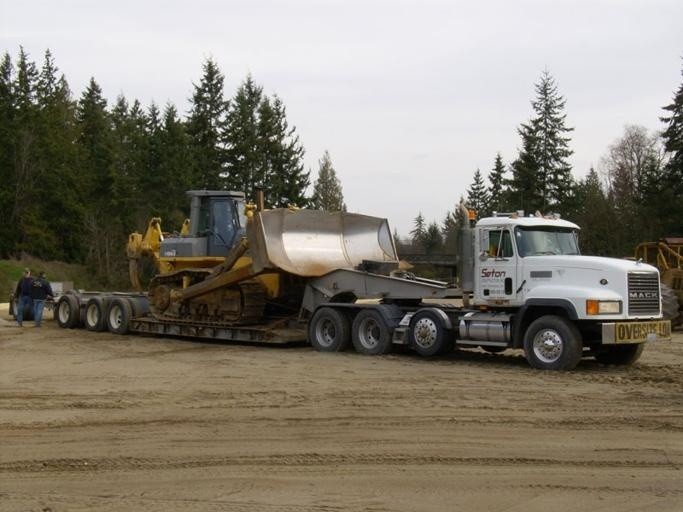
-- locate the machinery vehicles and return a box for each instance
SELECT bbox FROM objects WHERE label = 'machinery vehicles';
[124,190,414,327]
[624,236,683,330]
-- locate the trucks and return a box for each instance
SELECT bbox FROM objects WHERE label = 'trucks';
[9,203,671,370]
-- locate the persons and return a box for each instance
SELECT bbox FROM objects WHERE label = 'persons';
[30,271,55,328]
[10,267,33,328]
